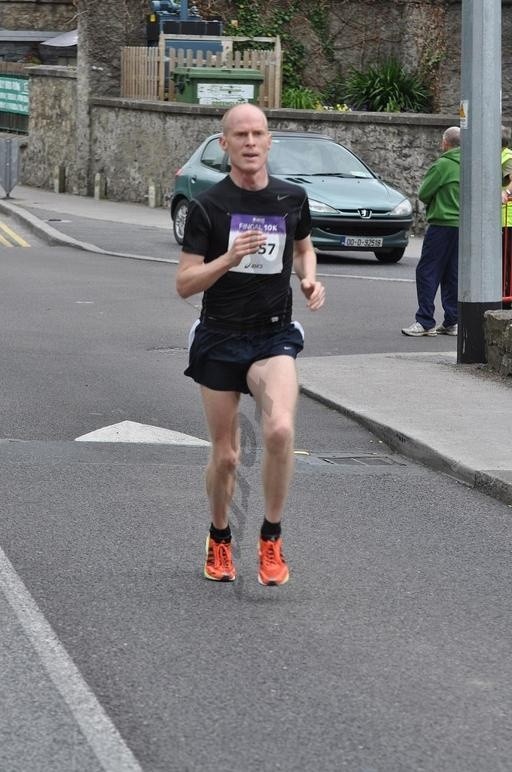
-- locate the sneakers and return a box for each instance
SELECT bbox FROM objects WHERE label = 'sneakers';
[257,535,290,587]
[401,321,438,337]
[436,324,457,335]
[203,531,236,582]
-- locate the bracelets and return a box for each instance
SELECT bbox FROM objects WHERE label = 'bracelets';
[506,189,512,197]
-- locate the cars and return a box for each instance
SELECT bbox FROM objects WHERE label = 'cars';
[170,129,415,265]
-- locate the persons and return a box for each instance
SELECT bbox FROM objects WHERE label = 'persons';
[176,104,327,586]
[500,122,512,296]
[400,126,461,337]
